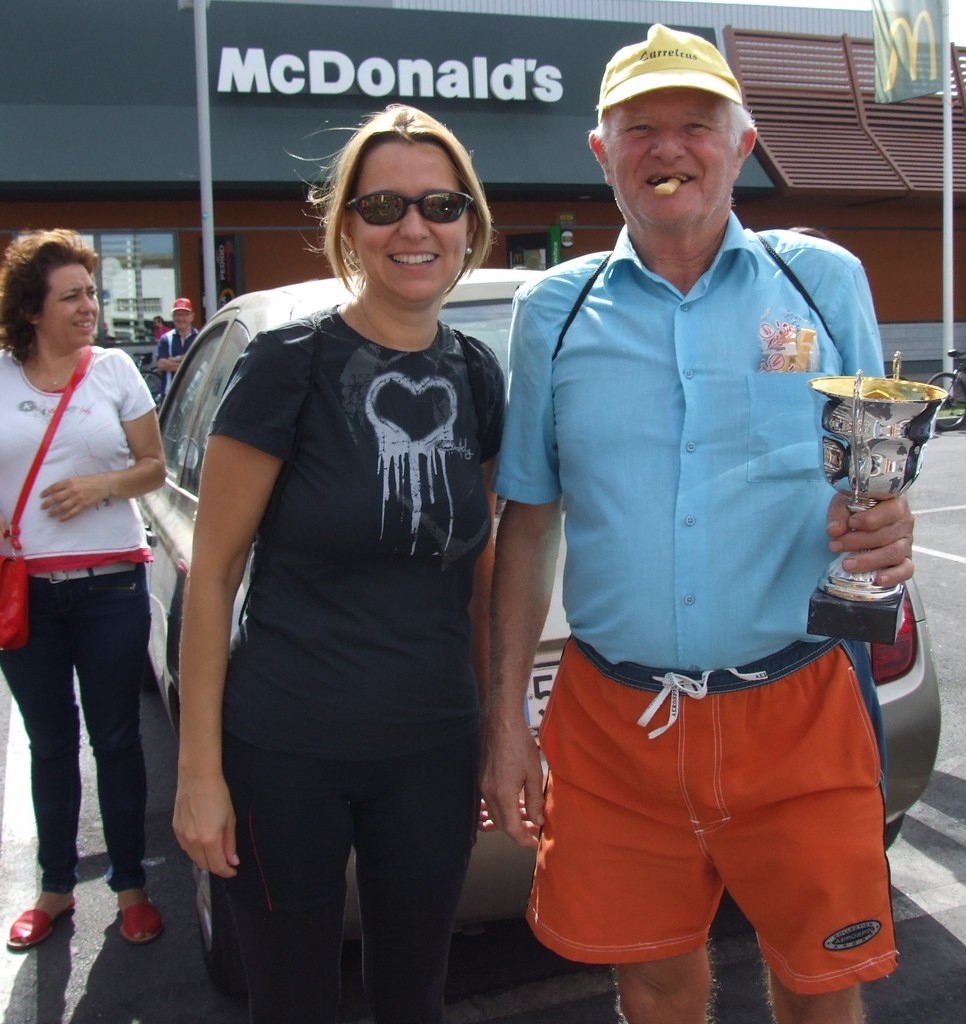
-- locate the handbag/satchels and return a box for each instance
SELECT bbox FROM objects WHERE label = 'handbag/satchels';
[0,550,30,651]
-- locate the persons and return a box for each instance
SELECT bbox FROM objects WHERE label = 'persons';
[172,108,505,1024]
[0,230,196,950]
[480,23,914,1024]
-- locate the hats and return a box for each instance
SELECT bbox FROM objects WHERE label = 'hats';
[597,23,742,125]
[171,298,192,312]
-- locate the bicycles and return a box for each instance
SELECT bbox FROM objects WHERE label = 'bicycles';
[925,347,966,431]
[131,352,164,410]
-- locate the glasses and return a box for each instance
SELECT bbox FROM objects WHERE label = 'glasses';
[344,190,475,226]
[173,312,192,316]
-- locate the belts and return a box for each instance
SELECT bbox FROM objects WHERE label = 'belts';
[30,561,137,584]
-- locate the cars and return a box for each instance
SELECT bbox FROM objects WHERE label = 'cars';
[132,263,944,991]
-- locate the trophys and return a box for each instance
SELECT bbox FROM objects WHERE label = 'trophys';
[808,351,948,645]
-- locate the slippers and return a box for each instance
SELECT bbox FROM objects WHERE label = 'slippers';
[7,892,76,951]
[120,888,165,945]
